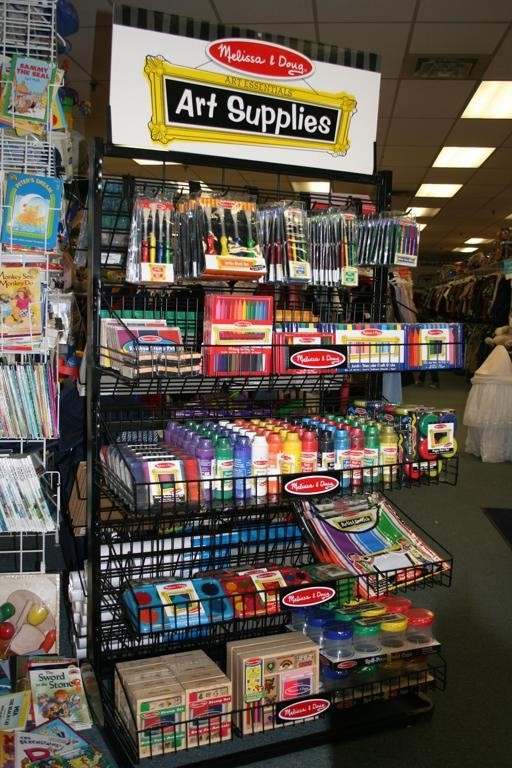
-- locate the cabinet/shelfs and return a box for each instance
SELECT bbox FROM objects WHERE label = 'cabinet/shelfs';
[82,137,465,768]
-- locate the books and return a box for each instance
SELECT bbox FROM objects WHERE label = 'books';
[1,362,59,439]
[1,170,64,355]
[69,462,126,537]
[0,452,63,531]
[2,53,67,137]
[0,655,108,768]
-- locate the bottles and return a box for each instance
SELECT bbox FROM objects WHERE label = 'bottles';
[164,413,399,502]
[290,596,437,696]
